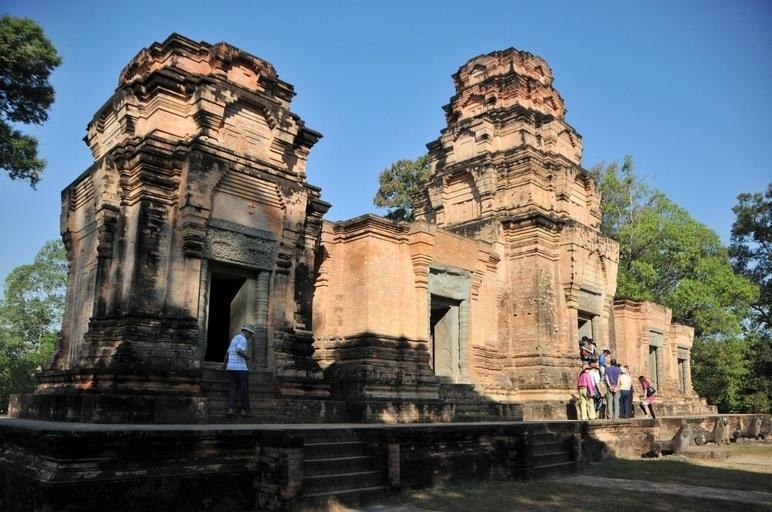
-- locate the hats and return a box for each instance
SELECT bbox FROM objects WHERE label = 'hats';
[241,322,257,335]
[582,364,592,370]
[621,368,628,374]
[602,348,612,354]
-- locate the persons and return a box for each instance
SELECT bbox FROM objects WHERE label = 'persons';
[223,324,256,416]
[577,335,655,420]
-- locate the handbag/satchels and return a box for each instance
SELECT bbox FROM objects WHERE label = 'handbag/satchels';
[588,353,599,361]
[647,387,655,397]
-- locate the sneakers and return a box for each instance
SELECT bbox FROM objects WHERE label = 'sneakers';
[227,407,237,415]
[240,409,250,417]
